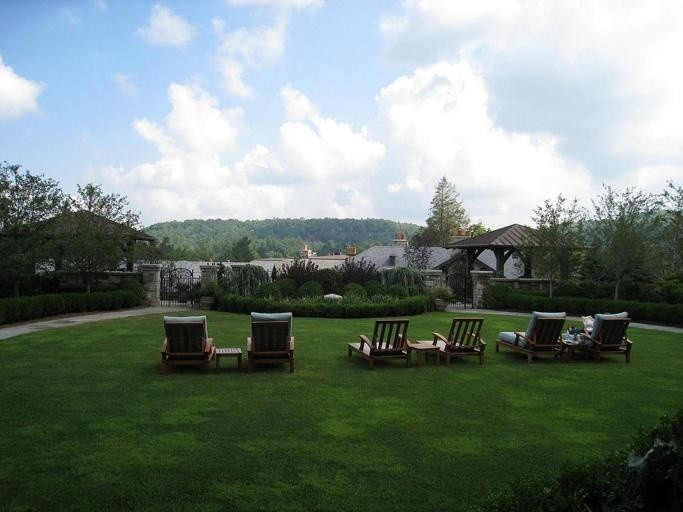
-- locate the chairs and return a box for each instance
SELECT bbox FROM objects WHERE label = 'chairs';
[432,314,491,368]
[495,310,568,363]
[245,311,296,377]
[574,310,635,362]
[157,310,214,370]
[347,318,411,367]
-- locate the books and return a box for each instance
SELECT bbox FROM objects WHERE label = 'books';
[581,315,595,331]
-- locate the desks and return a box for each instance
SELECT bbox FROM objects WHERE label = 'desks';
[557,337,589,361]
[215,346,241,372]
[411,339,439,367]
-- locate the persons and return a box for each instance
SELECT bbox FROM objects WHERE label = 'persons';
[566,324,592,336]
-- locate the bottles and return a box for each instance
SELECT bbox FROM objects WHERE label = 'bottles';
[571,332,587,345]
